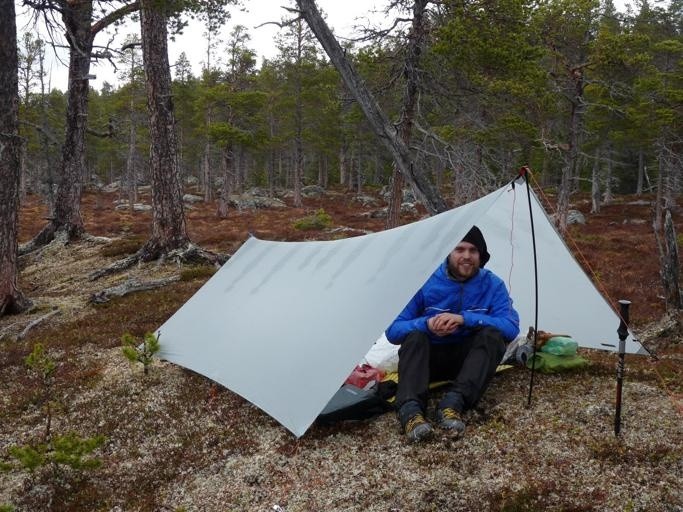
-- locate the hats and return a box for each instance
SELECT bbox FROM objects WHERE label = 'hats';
[461,225,490,270]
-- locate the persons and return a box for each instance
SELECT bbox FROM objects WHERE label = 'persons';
[385,225,520,442]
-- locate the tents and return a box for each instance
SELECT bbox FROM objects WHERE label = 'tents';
[141,176,650,440]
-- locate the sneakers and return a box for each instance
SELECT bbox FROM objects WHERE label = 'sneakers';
[403,411,434,443]
[434,405,466,434]
